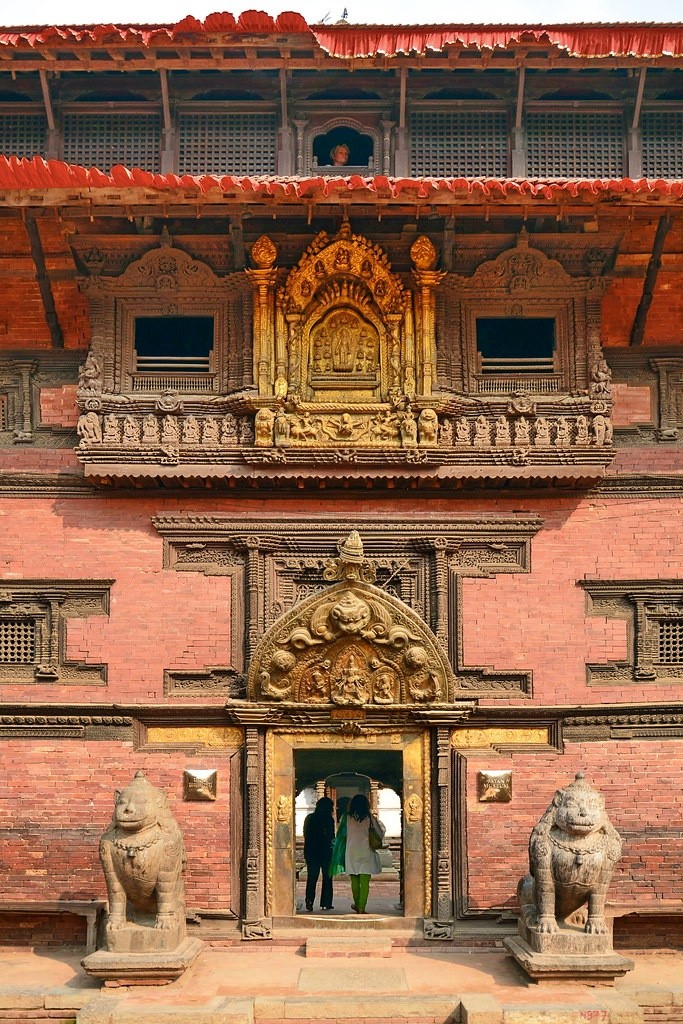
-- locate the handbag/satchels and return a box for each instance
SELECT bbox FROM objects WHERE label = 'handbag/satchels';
[369,815,381,849]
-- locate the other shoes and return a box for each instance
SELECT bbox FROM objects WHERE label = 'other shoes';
[358,910,369,914]
[351,905,358,912]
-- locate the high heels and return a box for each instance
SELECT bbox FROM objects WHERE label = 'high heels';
[322,906,334,910]
[306,899,313,911]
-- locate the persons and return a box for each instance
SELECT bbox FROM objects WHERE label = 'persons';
[380,405,415,440]
[106,414,158,437]
[164,415,214,437]
[286,303,301,314]
[303,797,335,911]
[330,144,350,166]
[336,249,351,268]
[343,662,362,699]
[315,261,325,276]
[376,281,385,293]
[537,418,586,438]
[329,794,386,914]
[362,261,371,276]
[496,416,532,439]
[277,408,313,439]
[335,329,351,364]
[440,415,490,443]
[222,417,253,438]
[301,280,310,294]
[338,414,353,435]
